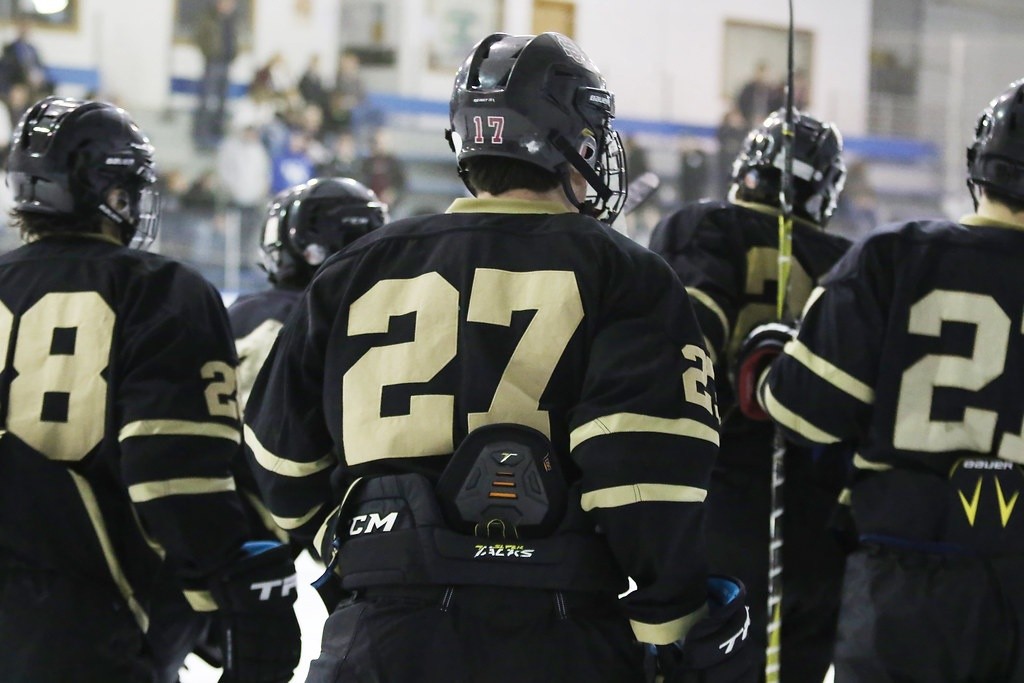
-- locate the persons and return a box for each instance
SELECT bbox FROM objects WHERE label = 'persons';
[255,51,401,205]
[221,169,391,683]
[0,92,301,683]
[0,19,56,126]
[191,0,236,152]
[240,29,749,683]
[617,55,881,230]
[648,108,859,683]
[728,81,1024,683]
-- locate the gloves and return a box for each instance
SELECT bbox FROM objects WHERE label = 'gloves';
[205,542,302,682]
[618,571,764,682]
[734,318,799,421]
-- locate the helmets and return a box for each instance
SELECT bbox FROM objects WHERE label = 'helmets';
[965,77,1023,202]
[6,95,160,250]
[259,176,387,285]
[730,109,845,228]
[445,31,627,226]
